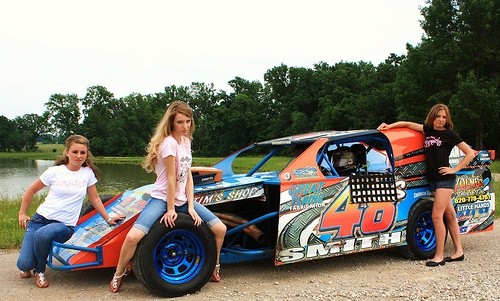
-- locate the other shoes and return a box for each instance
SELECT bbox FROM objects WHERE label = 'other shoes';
[426,258,445,267]
[19,270,32,278]
[35,271,49,288]
[444,253,464,261]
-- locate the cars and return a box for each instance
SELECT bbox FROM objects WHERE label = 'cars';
[45,127,496,298]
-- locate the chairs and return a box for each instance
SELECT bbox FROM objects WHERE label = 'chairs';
[350,143,368,175]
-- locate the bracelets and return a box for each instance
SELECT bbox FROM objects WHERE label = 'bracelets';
[107,218,111,222]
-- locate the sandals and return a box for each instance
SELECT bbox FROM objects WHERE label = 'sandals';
[211,264,221,282]
[110,267,127,293]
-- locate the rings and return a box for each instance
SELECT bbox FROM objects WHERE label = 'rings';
[24,221,26,222]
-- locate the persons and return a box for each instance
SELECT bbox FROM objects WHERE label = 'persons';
[377,104,476,267]
[110,101,227,293]
[16,135,126,288]
[332,146,354,169]
